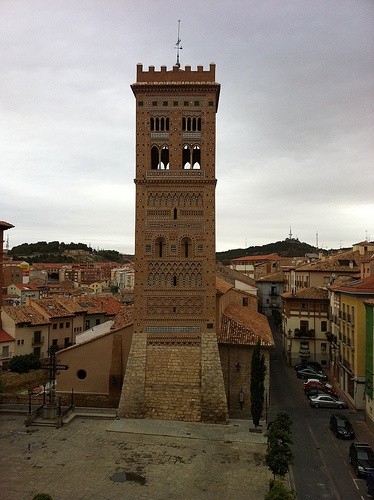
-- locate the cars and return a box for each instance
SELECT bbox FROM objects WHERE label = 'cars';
[294,361,347,410]
[330,414,356,441]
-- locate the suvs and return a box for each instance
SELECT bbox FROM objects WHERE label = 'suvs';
[349,441,374,479]
